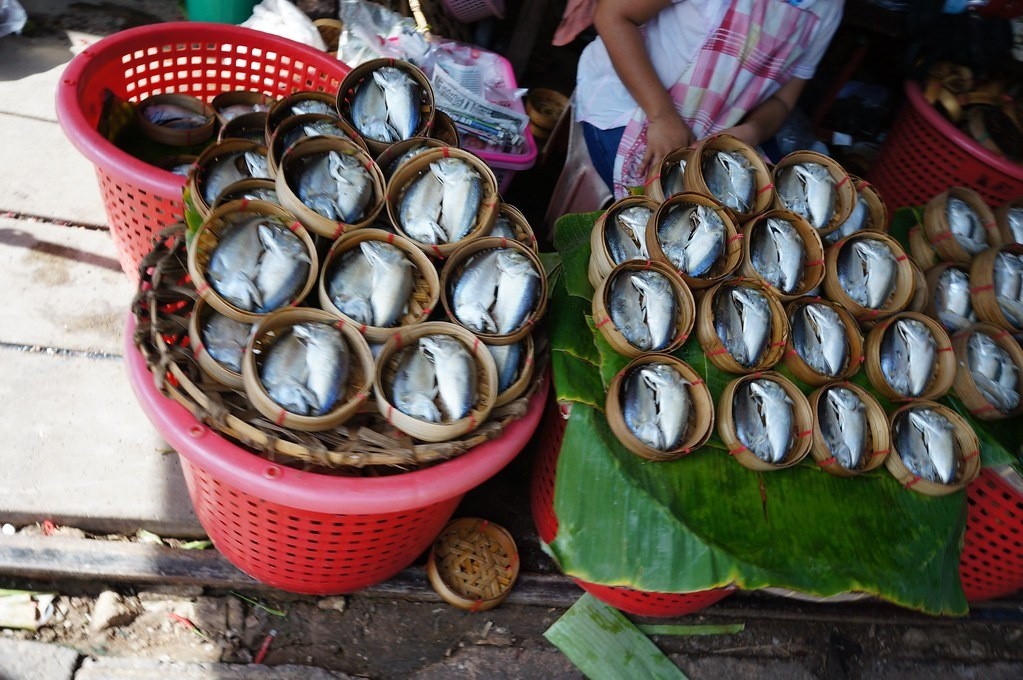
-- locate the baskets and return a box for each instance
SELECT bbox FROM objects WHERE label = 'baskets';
[531,302,744,617]
[52,22,362,289]
[446,0,505,23]
[122,219,552,599]
[131,55,1023,497]
[927,392,1022,601]
[425,517,520,610]
[379,30,538,202]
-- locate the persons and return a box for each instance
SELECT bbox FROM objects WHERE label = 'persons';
[572,0,849,215]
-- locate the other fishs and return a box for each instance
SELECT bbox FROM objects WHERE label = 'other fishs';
[118,52,1023,485]
